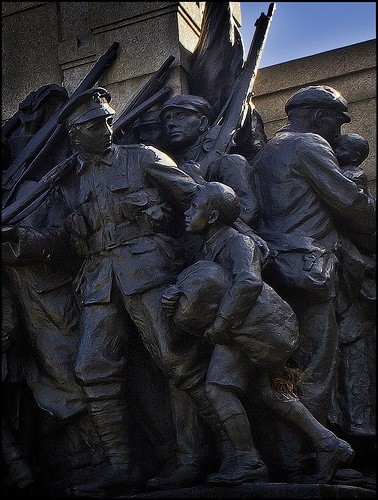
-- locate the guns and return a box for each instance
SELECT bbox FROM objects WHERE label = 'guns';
[201,1,276,181]
[117,54,175,121]
[0,40,120,211]
[0,86,173,222]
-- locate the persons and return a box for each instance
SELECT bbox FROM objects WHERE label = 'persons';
[0,82,378,495]
